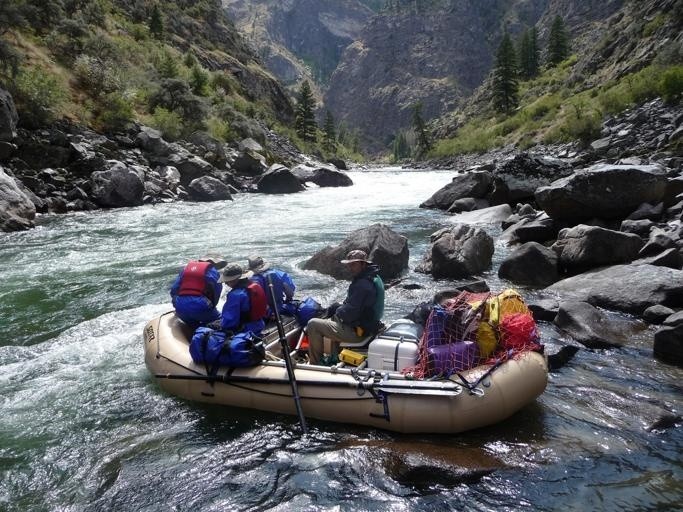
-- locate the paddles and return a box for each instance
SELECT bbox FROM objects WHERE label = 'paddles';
[154,372,464,397]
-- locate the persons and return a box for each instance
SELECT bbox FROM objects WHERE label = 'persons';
[168,256,227,330]
[244,254,296,324]
[303,248,385,366]
[205,262,268,338]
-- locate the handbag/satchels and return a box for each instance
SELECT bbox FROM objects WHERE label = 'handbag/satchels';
[421,287,542,379]
[188,321,266,368]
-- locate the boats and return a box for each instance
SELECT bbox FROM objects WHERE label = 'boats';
[142,306,550,433]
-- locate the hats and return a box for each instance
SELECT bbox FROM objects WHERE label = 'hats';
[242,254,270,274]
[216,262,254,283]
[339,249,375,266]
[197,255,229,270]
